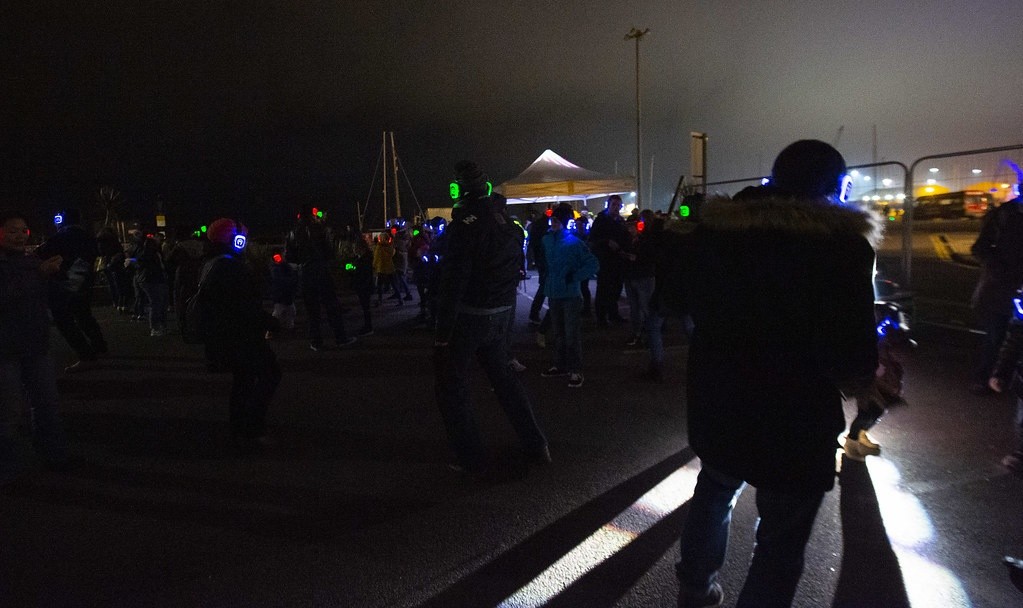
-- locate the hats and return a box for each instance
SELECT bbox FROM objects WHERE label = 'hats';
[207,218,248,246]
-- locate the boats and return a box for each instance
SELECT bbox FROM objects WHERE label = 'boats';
[32,224,147,274]
[930,233,984,284]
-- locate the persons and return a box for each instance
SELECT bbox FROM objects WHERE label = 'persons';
[939,235,982,268]
[417,160,554,487]
[527,187,710,388]
[843,300,903,464]
[0,210,64,486]
[343,217,421,340]
[33,207,357,450]
[970,183,1023,482]
[677,139,884,608]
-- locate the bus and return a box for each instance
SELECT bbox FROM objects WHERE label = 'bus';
[913,190,993,219]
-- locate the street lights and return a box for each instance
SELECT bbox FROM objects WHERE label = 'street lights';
[622,28,653,210]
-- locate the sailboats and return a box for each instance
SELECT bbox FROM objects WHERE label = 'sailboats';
[360,131,429,242]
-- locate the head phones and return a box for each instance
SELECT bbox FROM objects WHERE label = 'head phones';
[54,209,64,224]
[450,181,492,199]
[391,228,396,234]
[413,226,420,236]
[679,205,689,217]
[345,263,356,270]
[761,173,854,203]
[545,203,552,217]
[273,248,282,263]
[637,220,645,231]
[298,208,317,219]
[233,218,247,252]
[317,210,323,217]
[439,218,445,231]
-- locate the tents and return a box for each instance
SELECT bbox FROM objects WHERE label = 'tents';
[491,148,637,218]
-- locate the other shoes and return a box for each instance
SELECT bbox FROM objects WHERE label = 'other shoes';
[1001,555,1022,573]
[598,315,614,328]
[1,477,46,497]
[112,303,148,321]
[528,313,541,324]
[510,359,527,373]
[339,335,357,347]
[149,326,166,337]
[310,341,321,353]
[540,366,588,387]
[447,459,480,476]
[677,581,725,608]
[372,291,413,310]
[529,447,556,467]
[969,383,992,398]
[358,325,374,337]
[64,348,111,374]
[1003,448,1021,473]
[625,332,640,346]
